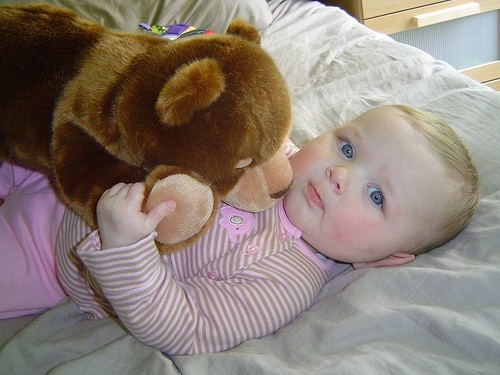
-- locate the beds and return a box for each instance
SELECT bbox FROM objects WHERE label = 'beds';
[0,0,500,374]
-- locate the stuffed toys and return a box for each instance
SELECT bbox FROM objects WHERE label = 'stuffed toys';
[1,3,295,254]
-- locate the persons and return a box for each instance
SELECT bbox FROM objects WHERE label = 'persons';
[0,104,480,356]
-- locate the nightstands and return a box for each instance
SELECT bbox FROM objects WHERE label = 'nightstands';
[323,0,500,92]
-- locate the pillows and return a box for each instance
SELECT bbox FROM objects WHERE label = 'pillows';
[57,1,270,30]
